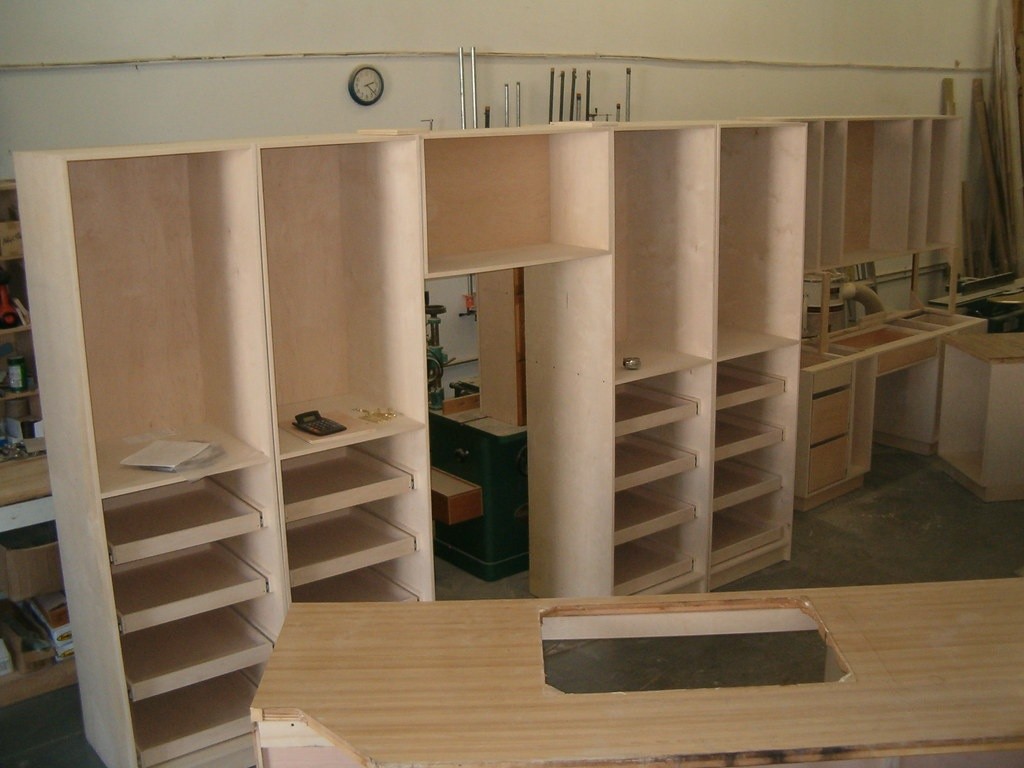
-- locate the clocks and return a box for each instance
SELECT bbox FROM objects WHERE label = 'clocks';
[348,64,384,106]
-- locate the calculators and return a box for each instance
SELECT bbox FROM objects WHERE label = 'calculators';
[292,410,346,436]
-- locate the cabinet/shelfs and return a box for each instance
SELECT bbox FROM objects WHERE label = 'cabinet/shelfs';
[475,267,527,427]
[524,121,718,596]
[242,134,434,609]
[794,113,988,515]
[698,119,810,593]
[0,179,77,709]
[11,139,288,768]
[934,332,1024,503]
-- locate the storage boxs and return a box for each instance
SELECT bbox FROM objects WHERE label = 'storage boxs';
[6,414,44,457]
[48,636,75,664]
[0,520,64,603]
[0,599,55,675]
[32,592,70,628]
[30,601,73,647]
[0,638,15,676]
